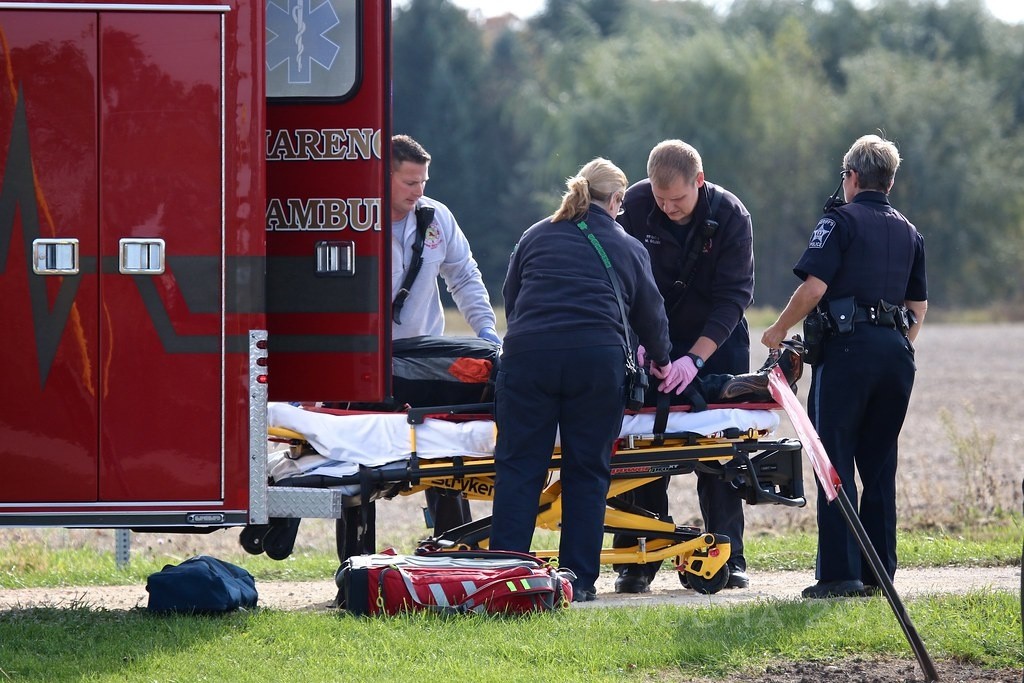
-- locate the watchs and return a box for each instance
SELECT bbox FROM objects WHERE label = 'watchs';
[685,353,705,371]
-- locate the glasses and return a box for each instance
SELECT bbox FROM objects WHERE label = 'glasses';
[840,171,849,179]
[616,198,626,215]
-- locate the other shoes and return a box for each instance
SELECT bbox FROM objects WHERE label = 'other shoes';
[802,580,866,598]
[725,566,749,589]
[573,586,596,601]
[615,565,648,593]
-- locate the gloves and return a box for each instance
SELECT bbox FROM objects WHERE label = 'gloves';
[658,356,698,395]
[637,345,672,379]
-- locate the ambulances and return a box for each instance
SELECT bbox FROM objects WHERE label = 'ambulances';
[0,0,393,535]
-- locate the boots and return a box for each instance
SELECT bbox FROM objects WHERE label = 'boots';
[717,333,805,401]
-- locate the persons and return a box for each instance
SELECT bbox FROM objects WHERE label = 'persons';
[335,134,503,565]
[762,128,928,598]
[489,157,673,602]
[613,139,756,596]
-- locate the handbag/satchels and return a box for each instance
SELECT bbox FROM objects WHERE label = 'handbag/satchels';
[626,323,648,411]
[145,556,258,613]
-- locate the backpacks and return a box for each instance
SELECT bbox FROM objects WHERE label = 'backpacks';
[327,549,577,617]
